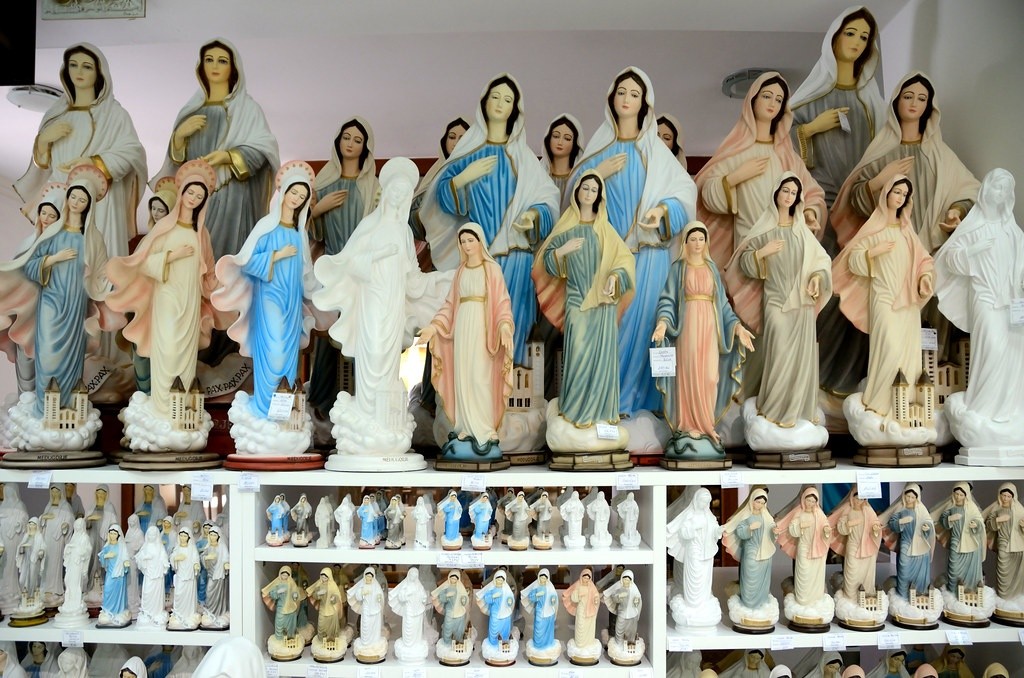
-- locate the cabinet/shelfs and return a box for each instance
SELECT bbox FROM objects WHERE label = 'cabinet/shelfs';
[0,469,1024,678]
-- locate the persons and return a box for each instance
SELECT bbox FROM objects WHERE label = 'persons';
[1,6,1024,677]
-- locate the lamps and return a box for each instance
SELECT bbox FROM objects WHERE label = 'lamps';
[5,84,64,114]
[721,67,782,99]
